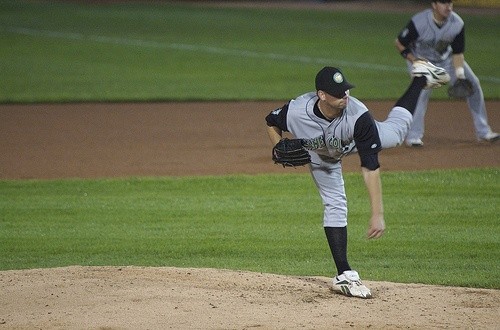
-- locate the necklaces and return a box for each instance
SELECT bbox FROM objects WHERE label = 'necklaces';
[432,14,450,25]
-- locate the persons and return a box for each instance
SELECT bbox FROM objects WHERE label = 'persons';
[265,61,451,299]
[394,0,500,148]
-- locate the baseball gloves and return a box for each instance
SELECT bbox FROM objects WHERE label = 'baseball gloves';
[271,137,312,169]
[448,77,474,98]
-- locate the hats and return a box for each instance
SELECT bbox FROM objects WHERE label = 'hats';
[438,0,451,3]
[315,66,355,95]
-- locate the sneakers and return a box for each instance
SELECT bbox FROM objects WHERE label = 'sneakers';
[411,60,451,88]
[406,138,423,148]
[477,132,500,143]
[332,270,371,299]
